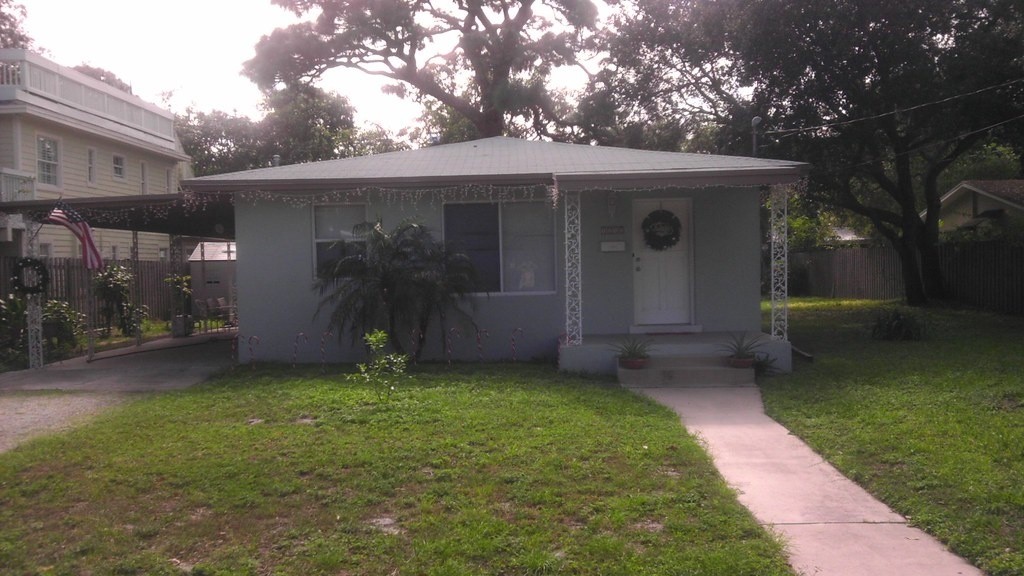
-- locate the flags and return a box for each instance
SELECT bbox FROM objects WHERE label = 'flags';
[49,201,103,269]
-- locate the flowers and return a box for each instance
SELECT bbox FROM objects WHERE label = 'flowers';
[641,210,682,251]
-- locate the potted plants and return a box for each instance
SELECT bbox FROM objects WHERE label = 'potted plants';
[605,332,663,369]
[712,330,769,368]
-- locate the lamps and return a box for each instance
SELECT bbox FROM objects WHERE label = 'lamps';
[607,190,618,217]
